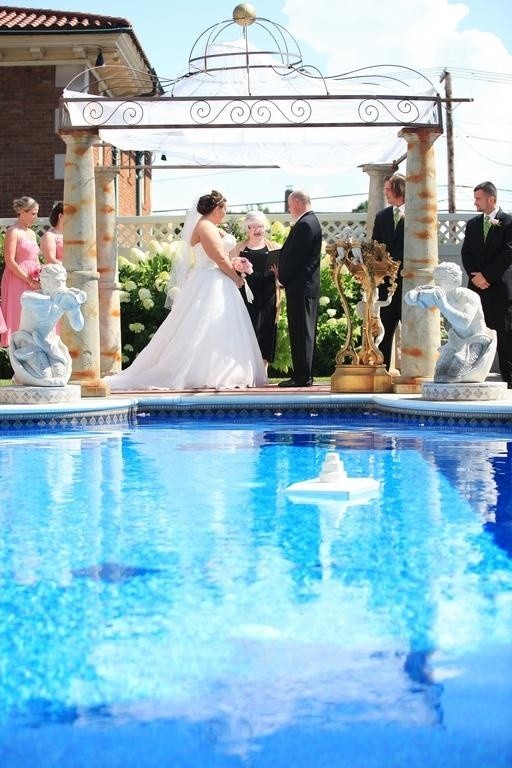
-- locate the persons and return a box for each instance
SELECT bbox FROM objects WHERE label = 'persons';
[271,191,320,386]
[41,202,63,333]
[8,264,88,386]
[0,196,40,350]
[404,263,497,383]
[101,189,266,390]
[372,174,405,371]
[229,210,282,379]
[461,181,510,386]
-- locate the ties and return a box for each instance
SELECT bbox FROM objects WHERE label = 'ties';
[393,208,400,231]
[483,216,491,243]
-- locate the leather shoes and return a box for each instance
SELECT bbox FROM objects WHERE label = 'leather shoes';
[278,377,312,387]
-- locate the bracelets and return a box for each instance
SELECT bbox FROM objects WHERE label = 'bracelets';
[235,275,240,283]
[28,278,33,286]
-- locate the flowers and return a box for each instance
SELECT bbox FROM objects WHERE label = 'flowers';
[231,255,254,289]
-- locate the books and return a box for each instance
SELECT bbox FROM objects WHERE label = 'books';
[239,247,280,272]
